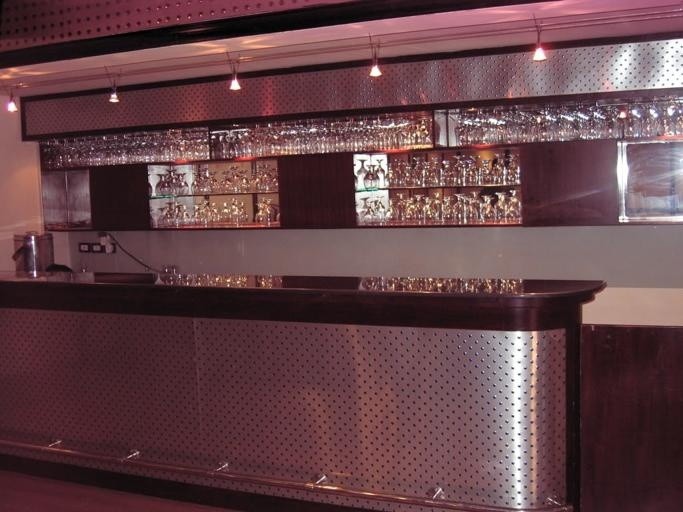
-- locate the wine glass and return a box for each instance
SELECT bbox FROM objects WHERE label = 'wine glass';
[456,93,683,147]
[39,108,433,170]
[354,155,522,226]
[147,162,279,228]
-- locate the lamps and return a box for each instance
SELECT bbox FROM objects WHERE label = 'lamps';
[369,43,383,76]
[533,28,546,61]
[8,86,18,112]
[109,74,119,102]
[229,61,241,90]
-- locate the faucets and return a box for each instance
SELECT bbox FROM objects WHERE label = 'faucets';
[12,231,41,271]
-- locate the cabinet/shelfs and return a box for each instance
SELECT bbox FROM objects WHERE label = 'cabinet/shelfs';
[352,145,523,295]
[147,158,283,289]
[42,167,93,231]
